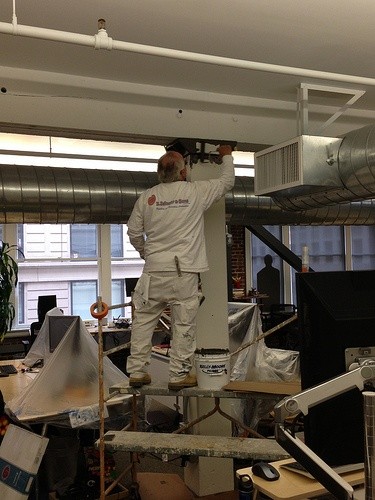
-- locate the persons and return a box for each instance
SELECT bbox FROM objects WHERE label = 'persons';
[127,142,236,390]
[256,254,280,311]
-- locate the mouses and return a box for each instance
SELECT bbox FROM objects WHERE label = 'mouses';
[0,373,9,377]
[252,462,280,481]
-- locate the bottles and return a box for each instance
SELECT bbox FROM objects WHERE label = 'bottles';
[302,246,309,273]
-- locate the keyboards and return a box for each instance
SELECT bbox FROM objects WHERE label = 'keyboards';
[0,365,17,374]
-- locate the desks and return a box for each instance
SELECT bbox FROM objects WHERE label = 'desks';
[236,458,365,500]
[0,359,141,423]
[87,326,131,334]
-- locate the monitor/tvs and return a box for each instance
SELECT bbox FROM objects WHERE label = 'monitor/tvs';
[48,316,80,353]
[296,269,375,470]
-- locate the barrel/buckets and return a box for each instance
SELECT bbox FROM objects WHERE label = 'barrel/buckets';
[195,349,230,391]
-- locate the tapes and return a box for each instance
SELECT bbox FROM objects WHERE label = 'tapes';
[90,302,108,318]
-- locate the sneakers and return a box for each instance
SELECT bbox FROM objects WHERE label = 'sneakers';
[168,374,197,388]
[129,373,152,386]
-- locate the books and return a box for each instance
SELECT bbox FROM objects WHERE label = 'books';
[0,424,49,500]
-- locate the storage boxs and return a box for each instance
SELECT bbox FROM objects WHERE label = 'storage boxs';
[0,424,50,500]
